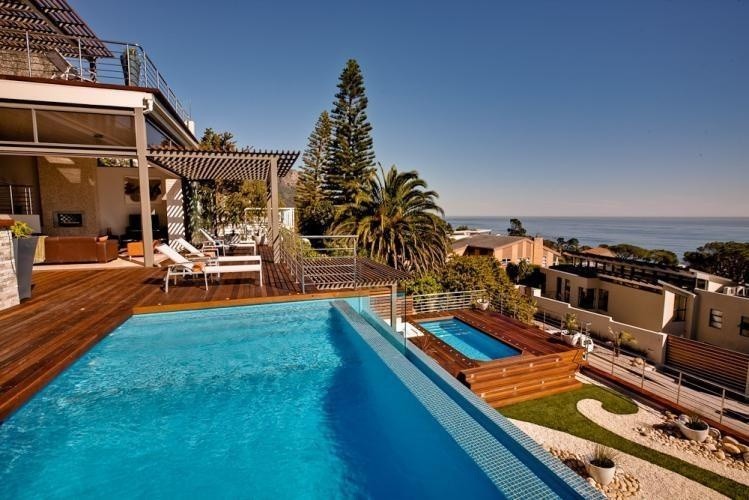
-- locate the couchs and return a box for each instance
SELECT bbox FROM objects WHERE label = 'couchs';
[35,234,120,265]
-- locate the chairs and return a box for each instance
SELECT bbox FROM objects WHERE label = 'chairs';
[127,226,270,293]
[45,45,97,82]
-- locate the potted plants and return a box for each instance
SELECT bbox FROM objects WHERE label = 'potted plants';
[680,406,712,444]
[119,47,141,86]
[9,220,38,300]
[581,441,622,485]
[560,310,582,346]
